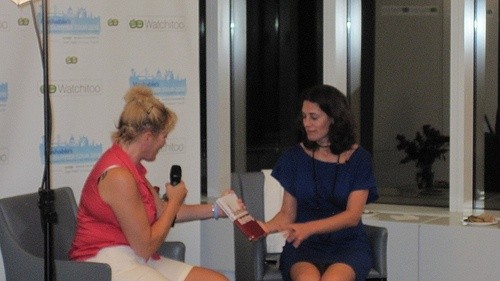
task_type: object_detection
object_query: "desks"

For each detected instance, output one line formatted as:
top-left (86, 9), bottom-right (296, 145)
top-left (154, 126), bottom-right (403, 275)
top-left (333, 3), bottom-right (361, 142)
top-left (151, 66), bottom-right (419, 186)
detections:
top-left (360, 210), bottom-right (500, 281)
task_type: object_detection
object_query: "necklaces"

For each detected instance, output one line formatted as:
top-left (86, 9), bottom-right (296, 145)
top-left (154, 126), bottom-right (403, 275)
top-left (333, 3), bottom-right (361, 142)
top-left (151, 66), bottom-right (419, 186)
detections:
top-left (312, 146), bottom-right (341, 208)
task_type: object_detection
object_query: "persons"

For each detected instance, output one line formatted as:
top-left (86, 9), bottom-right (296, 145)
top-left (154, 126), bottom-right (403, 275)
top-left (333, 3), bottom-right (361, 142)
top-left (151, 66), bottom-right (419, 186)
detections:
top-left (67, 85), bottom-right (246, 281)
top-left (254, 85), bottom-right (380, 281)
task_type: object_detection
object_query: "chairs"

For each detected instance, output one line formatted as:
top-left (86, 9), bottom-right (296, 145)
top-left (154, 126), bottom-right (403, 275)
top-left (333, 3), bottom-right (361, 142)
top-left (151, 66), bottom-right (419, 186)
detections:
top-left (231, 169), bottom-right (388, 281)
top-left (0, 186), bottom-right (186, 281)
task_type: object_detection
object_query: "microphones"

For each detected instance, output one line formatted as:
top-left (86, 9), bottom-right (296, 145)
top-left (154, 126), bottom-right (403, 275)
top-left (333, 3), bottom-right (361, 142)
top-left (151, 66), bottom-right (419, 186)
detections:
top-left (169, 165), bottom-right (182, 227)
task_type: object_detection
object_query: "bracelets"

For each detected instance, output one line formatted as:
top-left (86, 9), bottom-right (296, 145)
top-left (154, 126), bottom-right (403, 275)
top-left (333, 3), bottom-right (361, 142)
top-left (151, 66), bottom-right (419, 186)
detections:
top-left (213, 205), bottom-right (219, 219)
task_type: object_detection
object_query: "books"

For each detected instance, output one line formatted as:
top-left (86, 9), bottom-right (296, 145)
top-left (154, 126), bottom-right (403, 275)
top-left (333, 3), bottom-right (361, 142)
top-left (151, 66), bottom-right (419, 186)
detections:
top-left (216, 193), bottom-right (266, 242)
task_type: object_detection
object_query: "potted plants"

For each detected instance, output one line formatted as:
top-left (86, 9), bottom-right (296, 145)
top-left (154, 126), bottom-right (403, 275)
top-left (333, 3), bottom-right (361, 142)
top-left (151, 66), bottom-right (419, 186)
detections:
top-left (396, 125), bottom-right (450, 188)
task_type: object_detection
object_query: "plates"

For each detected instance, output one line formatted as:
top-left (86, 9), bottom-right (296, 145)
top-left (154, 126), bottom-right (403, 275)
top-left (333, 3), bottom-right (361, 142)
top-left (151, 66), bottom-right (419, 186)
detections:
top-left (461, 218), bottom-right (498, 225)
top-left (362, 211), bottom-right (377, 216)
